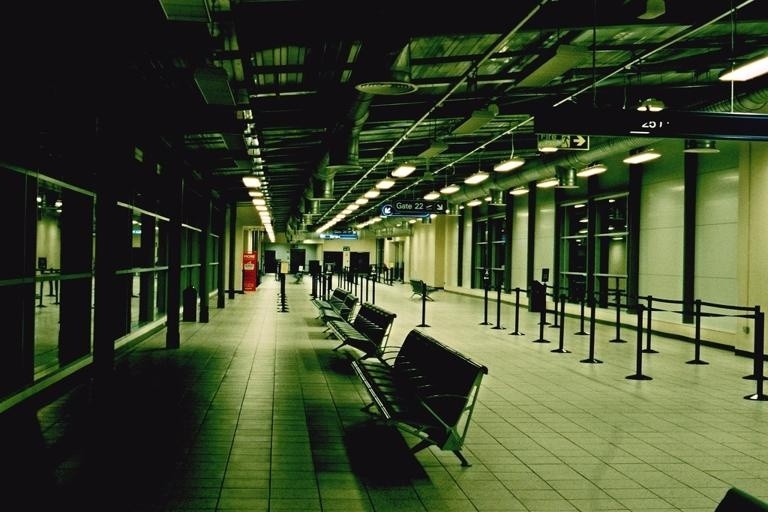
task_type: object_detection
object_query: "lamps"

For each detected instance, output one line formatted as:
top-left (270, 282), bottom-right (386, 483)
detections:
top-left (718, 49), bottom-right (768, 83)
top-left (249, 189), bottom-right (264, 197)
top-left (622, 150), bottom-right (662, 165)
top-left (464, 172), bottom-right (489, 185)
top-left (375, 177), bottom-right (396, 190)
top-left (424, 190), bottom-right (441, 201)
top-left (252, 198), bottom-right (276, 242)
top-left (509, 187), bottom-right (531, 196)
top-left (493, 157), bottom-right (525, 173)
top-left (537, 134), bottom-right (558, 152)
top-left (391, 163), bottom-right (417, 179)
top-left (242, 176), bottom-right (261, 188)
top-left (636, 98), bottom-right (666, 112)
top-left (439, 184), bottom-right (461, 195)
top-left (316, 189), bottom-right (381, 234)
top-left (575, 164), bottom-right (607, 178)
top-left (536, 178), bottom-right (560, 188)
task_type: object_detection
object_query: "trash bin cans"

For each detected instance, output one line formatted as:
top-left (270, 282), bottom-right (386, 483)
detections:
top-left (183, 285), bottom-right (197, 322)
top-left (532, 280), bottom-right (547, 312)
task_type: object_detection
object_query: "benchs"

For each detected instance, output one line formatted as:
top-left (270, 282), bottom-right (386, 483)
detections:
top-left (312, 279), bottom-right (489, 469)
